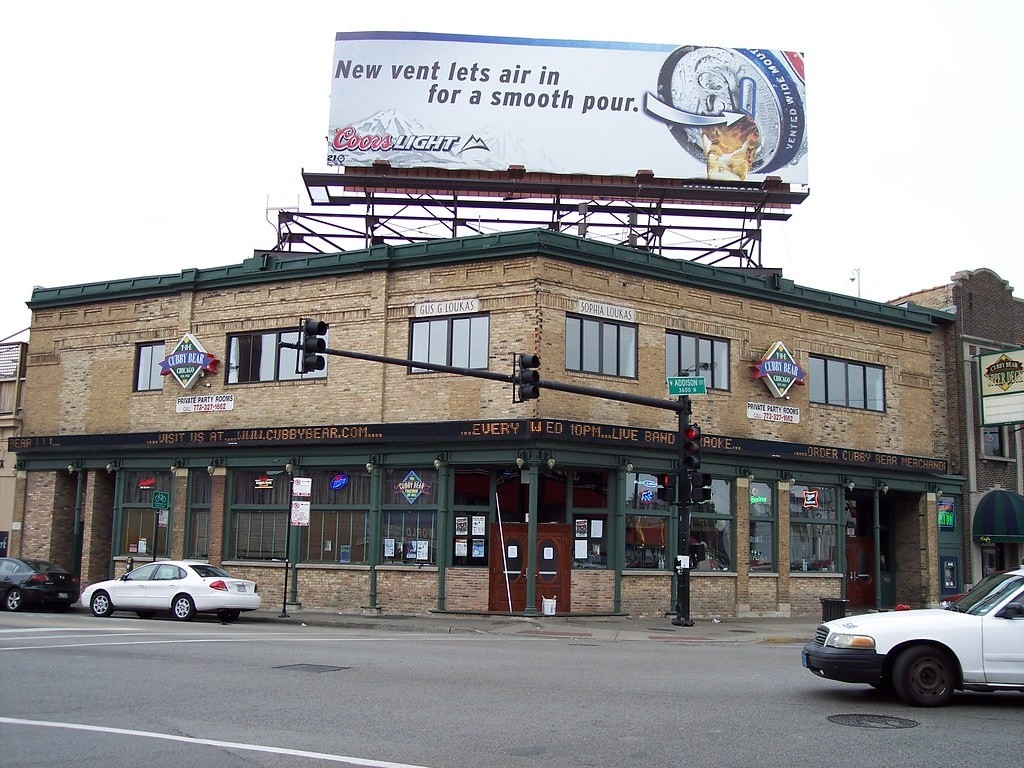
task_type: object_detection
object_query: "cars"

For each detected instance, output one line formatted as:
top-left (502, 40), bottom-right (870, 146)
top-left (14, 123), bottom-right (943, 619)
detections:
top-left (0, 557), bottom-right (80, 613)
top-left (79, 560), bottom-right (262, 622)
top-left (800, 565), bottom-right (1024, 707)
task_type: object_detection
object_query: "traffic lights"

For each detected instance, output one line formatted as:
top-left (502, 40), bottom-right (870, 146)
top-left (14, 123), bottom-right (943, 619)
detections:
top-left (295, 317), bottom-right (325, 374)
top-left (683, 424), bottom-right (702, 470)
top-left (689, 473), bottom-right (714, 504)
top-left (513, 352), bottom-right (540, 403)
top-left (655, 472), bottom-right (677, 503)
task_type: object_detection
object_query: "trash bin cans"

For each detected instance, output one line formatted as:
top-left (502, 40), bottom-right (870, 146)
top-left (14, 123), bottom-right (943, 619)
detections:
top-left (819, 598), bottom-right (850, 623)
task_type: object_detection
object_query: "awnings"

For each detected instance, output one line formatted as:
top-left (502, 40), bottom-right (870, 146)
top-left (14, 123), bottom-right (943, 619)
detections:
top-left (972, 490), bottom-right (1024, 544)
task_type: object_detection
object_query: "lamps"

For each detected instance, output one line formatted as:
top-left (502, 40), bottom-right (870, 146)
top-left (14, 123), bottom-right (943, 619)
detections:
top-left (106, 460), bottom-right (118, 474)
top-left (372, 158), bottom-right (393, 176)
top-left (11, 463), bottom-right (23, 476)
top-left (365, 457), bottom-right (377, 474)
top-left (286, 457), bottom-right (297, 476)
top-left (170, 458), bottom-right (182, 476)
top-left (743, 468), bottom-right (754, 484)
top-left (434, 453), bottom-right (445, 470)
top-left (925, 482), bottom-right (942, 499)
top-left (785, 473), bottom-right (796, 488)
top-left (207, 457), bottom-right (219, 477)
top-left (634, 169), bottom-right (655, 184)
top-left (843, 476), bottom-right (856, 491)
top-left (544, 450), bottom-right (556, 469)
top-left (506, 164), bottom-right (526, 181)
top-left (878, 480), bottom-right (889, 494)
top-left (622, 456), bottom-right (634, 474)
top-left (516, 451), bottom-right (529, 469)
top-left (67, 460), bottom-right (80, 474)
top-left (762, 177), bottom-right (783, 190)
